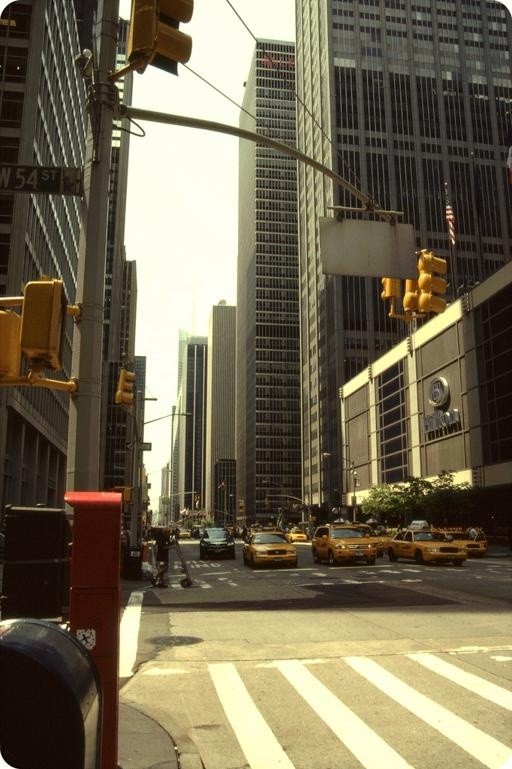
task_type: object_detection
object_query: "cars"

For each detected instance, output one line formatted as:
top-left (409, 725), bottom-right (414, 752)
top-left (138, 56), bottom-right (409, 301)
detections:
top-left (311, 520), bottom-right (377, 567)
top-left (348, 522), bottom-right (385, 559)
top-left (198, 527), bottom-right (236, 560)
top-left (284, 526), bottom-right (307, 544)
top-left (381, 520), bottom-right (468, 567)
top-left (242, 531), bottom-right (297, 569)
top-left (437, 531), bottom-right (486, 558)
top-left (173, 525), bottom-right (282, 544)
top-left (372, 528), bottom-right (393, 558)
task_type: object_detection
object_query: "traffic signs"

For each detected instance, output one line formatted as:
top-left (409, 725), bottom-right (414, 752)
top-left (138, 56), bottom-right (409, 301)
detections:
top-left (126, 442), bottom-right (152, 452)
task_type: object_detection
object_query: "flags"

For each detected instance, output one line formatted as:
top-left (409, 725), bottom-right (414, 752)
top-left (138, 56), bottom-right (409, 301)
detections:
top-left (445, 189), bottom-right (457, 245)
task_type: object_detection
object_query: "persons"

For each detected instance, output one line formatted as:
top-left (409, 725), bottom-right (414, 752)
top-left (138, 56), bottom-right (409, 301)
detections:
top-left (153, 527), bottom-right (174, 587)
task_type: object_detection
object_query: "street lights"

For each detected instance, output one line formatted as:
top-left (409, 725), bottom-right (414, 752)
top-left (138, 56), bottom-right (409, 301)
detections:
top-left (323, 452), bottom-right (356, 522)
top-left (139, 412), bottom-right (193, 551)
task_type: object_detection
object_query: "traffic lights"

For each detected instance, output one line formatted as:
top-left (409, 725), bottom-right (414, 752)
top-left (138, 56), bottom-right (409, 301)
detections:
top-left (419, 252), bottom-right (447, 316)
top-left (123, 487), bottom-right (132, 503)
top-left (115, 368), bottom-right (136, 404)
top-left (125, 0), bottom-right (194, 76)
top-left (20, 280), bottom-right (68, 372)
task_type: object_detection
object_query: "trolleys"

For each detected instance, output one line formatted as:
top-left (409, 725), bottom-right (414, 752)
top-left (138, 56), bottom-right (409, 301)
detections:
top-left (174, 541), bottom-right (200, 588)
